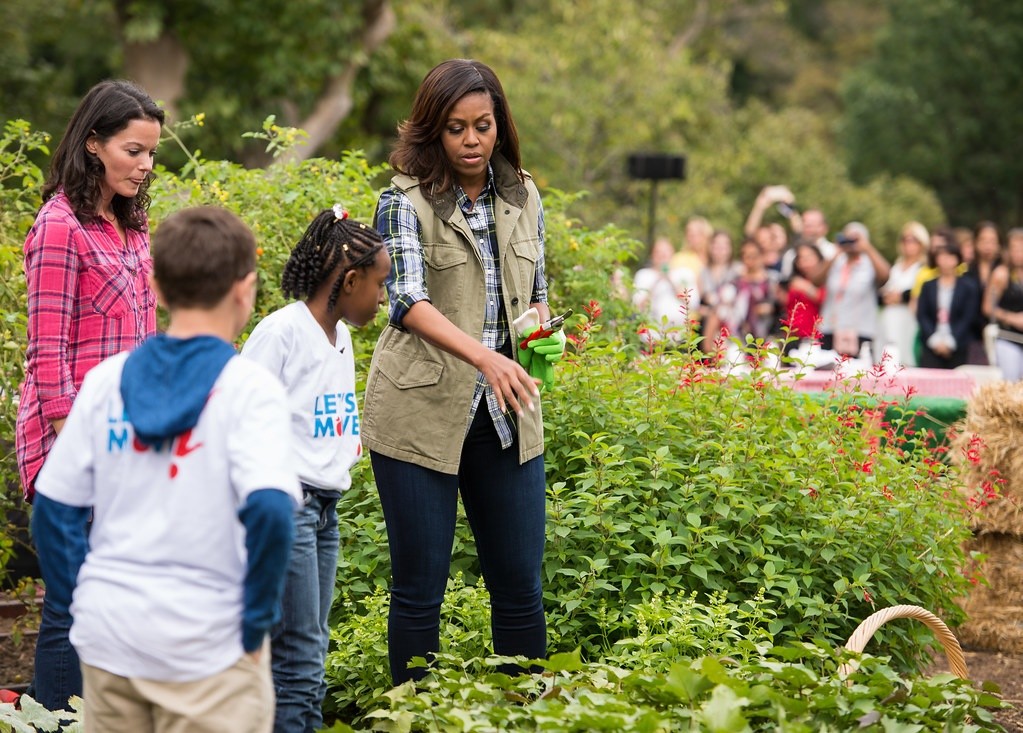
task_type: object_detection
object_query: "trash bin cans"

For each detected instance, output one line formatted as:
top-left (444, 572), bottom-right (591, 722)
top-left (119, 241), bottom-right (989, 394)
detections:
top-left (794, 392), bottom-right (872, 442)
top-left (861, 397), bottom-right (968, 467)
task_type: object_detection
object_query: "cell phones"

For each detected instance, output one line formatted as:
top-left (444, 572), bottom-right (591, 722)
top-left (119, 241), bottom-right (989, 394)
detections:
top-left (776, 203), bottom-right (794, 219)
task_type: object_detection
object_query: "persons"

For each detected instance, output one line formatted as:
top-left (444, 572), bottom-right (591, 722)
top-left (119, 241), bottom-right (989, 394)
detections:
top-left (361, 61), bottom-right (568, 706)
top-left (29, 204), bottom-right (305, 733)
top-left (575, 183), bottom-right (1023, 389)
top-left (15, 77), bottom-right (166, 733)
top-left (240, 209), bottom-right (391, 733)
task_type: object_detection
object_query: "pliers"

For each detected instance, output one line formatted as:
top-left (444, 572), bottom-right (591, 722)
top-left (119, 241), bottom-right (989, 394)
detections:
top-left (520, 308), bottom-right (573, 351)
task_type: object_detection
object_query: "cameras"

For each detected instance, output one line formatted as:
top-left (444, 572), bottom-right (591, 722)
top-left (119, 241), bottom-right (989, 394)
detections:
top-left (837, 233), bottom-right (855, 245)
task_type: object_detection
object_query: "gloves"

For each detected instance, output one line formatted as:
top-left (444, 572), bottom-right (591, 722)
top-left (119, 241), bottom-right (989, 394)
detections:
top-left (522, 319), bottom-right (566, 363)
top-left (512, 308), bottom-right (556, 393)
